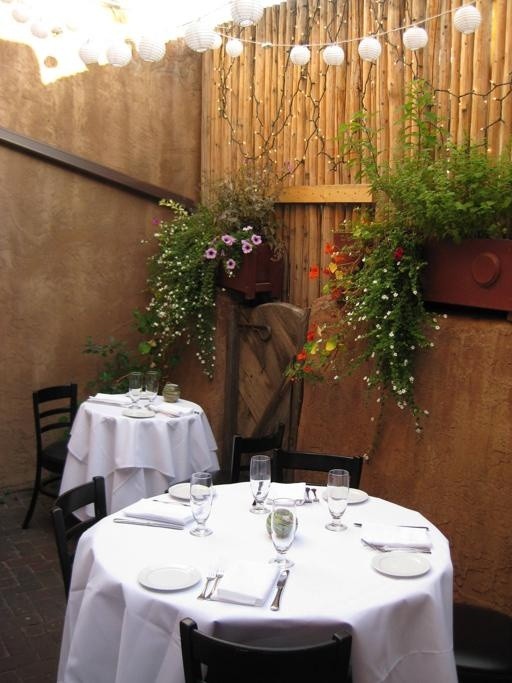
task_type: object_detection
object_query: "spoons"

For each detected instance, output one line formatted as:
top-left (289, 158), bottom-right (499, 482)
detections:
top-left (204, 569), bottom-right (224, 599)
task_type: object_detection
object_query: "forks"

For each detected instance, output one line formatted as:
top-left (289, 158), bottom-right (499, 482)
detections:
top-left (199, 571), bottom-right (214, 598)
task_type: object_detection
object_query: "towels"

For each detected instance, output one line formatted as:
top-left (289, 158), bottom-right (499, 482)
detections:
top-left (126, 498), bottom-right (194, 526)
top-left (88, 393), bottom-right (139, 407)
top-left (363, 523), bottom-right (432, 553)
top-left (210, 557), bottom-right (279, 606)
top-left (147, 399), bottom-right (192, 417)
top-left (266, 481), bottom-right (308, 508)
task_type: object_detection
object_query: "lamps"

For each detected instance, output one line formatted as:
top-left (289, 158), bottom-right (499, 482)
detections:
top-left (0, 0), bottom-right (481, 66)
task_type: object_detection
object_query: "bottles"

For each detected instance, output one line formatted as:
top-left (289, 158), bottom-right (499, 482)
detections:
top-left (164, 384), bottom-right (180, 403)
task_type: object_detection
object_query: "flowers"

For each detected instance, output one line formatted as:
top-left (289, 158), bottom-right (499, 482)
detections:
top-left (287, 75), bottom-right (512, 434)
top-left (145, 159), bottom-right (296, 381)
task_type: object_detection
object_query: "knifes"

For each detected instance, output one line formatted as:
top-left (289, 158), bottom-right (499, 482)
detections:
top-left (114, 519), bottom-right (182, 530)
top-left (272, 569), bottom-right (288, 609)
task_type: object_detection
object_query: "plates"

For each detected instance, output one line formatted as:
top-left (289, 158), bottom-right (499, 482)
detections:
top-left (373, 551), bottom-right (431, 576)
top-left (138, 564), bottom-right (200, 590)
top-left (322, 484), bottom-right (367, 502)
top-left (169, 481), bottom-right (213, 499)
top-left (123, 410), bottom-right (154, 419)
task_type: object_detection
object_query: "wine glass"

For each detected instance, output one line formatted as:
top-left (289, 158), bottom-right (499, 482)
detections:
top-left (129, 372), bottom-right (142, 409)
top-left (325, 469), bottom-right (350, 530)
top-left (146, 371), bottom-right (158, 407)
top-left (190, 472), bottom-right (213, 536)
top-left (271, 499), bottom-right (297, 564)
top-left (250, 455), bottom-right (269, 513)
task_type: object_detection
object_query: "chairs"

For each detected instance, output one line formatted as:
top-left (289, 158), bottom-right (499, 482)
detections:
top-left (21, 382), bottom-right (77, 529)
top-left (453, 602), bottom-right (512, 680)
top-left (52, 476), bottom-right (107, 605)
top-left (272, 449), bottom-right (363, 488)
top-left (230, 422), bottom-right (285, 483)
top-left (180, 615), bottom-right (353, 683)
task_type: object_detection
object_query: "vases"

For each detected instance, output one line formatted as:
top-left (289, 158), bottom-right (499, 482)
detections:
top-left (412, 237), bottom-right (512, 321)
top-left (217, 245), bottom-right (282, 303)
